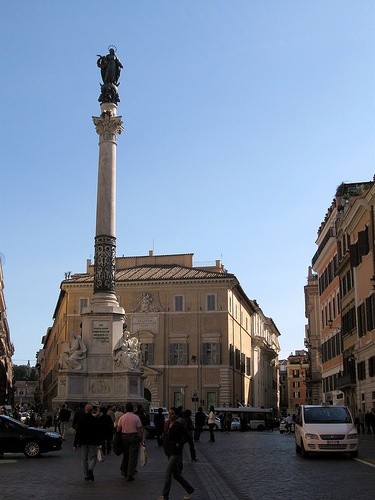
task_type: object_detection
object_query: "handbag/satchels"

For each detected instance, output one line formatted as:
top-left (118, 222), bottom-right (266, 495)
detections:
top-left (95, 446), bottom-right (105, 462)
top-left (139, 446), bottom-right (147, 467)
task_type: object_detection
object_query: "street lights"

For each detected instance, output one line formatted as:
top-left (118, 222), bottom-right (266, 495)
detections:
top-left (305, 340), bottom-right (321, 350)
top-left (328, 319), bottom-right (352, 336)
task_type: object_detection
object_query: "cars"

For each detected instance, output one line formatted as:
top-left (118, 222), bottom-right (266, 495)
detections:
top-left (295, 404), bottom-right (358, 458)
top-left (149, 408), bottom-right (169, 421)
top-left (280, 420), bottom-right (294, 434)
top-left (0, 414), bottom-right (62, 457)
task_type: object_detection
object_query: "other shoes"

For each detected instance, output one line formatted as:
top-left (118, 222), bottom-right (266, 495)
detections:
top-left (62, 438), bottom-right (65, 441)
top-left (208, 438), bottom-right (215, 442)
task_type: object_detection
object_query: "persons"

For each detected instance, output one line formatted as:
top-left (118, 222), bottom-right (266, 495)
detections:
top-left (96, 45), bottom-right (123, 87)
top-left (354, 408), bottom-right (375, 435)
top-left (0, 402), bottom-right (295, 500)
top-left (112, 332), bottom-right (141, 371)
top-left (58, 335), bottom-right (87, 369)
top-left (139, 292), bottom-right (150, 313)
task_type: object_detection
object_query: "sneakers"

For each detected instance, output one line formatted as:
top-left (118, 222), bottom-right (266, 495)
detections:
top-left (121, 469), bottom-right (138, 481)
top-left (158, 496), bottom-right (168, 500)
top-left (184, 490), bottom-right (194, 500)
top-left (85, 469), bottom-right (94, 481)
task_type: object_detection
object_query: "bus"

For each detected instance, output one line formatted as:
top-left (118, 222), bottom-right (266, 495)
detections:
top-left (207, 407), bottom-right (274, 431)
